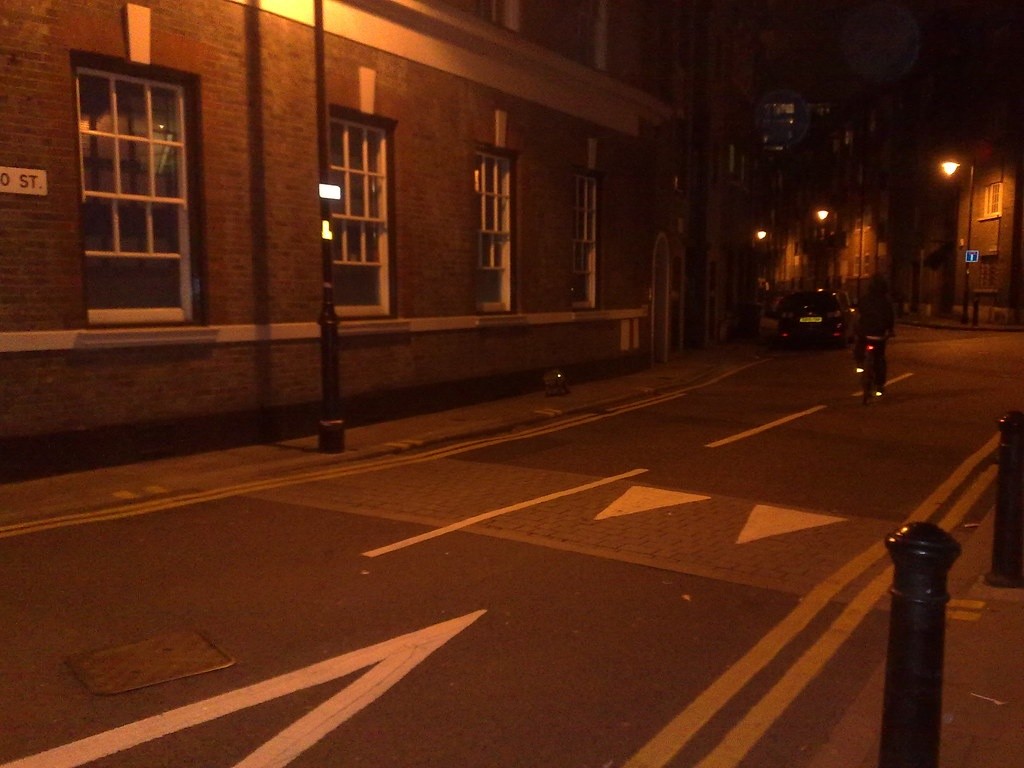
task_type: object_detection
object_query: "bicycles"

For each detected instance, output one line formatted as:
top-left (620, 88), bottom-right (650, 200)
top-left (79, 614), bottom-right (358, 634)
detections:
top-left (855, 333), bottom-right (884, 406)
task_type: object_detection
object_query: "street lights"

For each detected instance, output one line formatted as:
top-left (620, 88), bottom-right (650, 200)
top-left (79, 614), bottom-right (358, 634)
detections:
top-left (757, 226), bottom-right (775, 296)
top-left (815, 206), bottom-right (838, 288)
top-left (939, 159), bottom-right (974, 323)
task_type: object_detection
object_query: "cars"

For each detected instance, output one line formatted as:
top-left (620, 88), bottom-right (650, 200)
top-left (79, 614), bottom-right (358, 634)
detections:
top-left (776, 289), bottom-right (857, 348)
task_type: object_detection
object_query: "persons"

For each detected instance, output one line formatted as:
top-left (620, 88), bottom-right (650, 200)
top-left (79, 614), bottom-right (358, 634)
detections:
top-left (790, 271), bottom-right (842, 290)
top-left (857, 274), bottom-right (895, 393)
